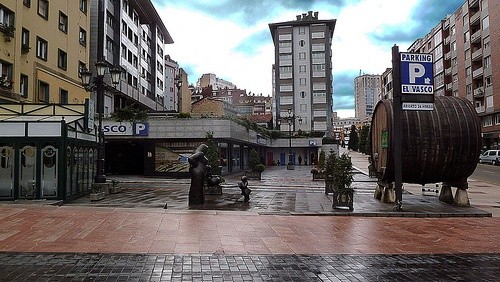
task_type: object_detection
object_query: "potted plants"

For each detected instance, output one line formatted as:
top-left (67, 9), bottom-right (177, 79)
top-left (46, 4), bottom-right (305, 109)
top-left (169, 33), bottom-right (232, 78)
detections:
top-left (90, 180), bottom-right (121, 201)
top-left (205, 131), bottom-right (225, 194)
top-left (368, 164), bottom-right (377, 178)
top-left (245, 148), bottom-right (265, 180)
top-left (313, 148), bottom-right (355, 211)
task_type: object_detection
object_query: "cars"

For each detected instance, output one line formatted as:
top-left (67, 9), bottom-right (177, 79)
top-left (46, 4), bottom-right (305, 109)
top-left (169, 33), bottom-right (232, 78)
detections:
top-left (478, 150), bottom-right (500, 166)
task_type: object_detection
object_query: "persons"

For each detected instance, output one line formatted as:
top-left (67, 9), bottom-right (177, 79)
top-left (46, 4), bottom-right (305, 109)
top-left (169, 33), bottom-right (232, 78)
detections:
top-left (298, 155), bottom-right (302, 165)
top-left (238, 176), bottom-right (251, 203)
top-left (187, 144), bottom-right (208, 204)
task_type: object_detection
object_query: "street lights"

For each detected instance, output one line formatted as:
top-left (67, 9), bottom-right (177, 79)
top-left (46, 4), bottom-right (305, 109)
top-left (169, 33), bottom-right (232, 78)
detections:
top-left (81, 56), bottom-right (123, 196)
top-left (277, 107), bottom-right (303, 170)
